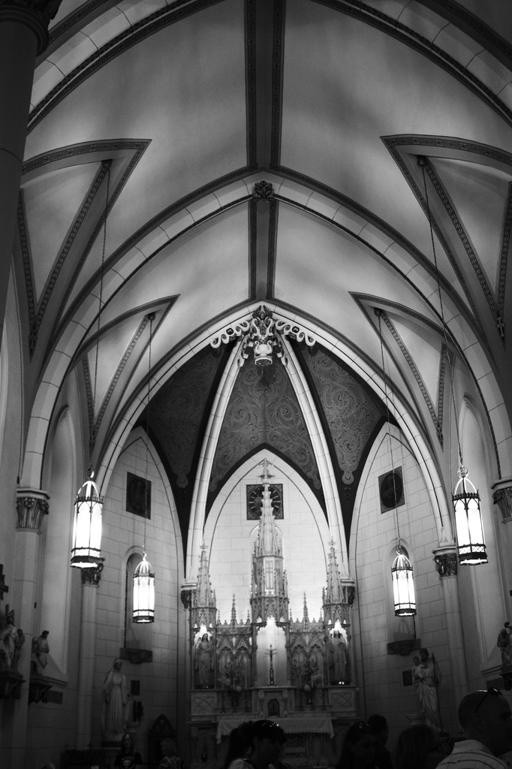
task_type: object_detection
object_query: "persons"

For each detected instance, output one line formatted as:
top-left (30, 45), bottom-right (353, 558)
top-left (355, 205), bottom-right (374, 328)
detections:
top-left (115, 734), bottom-right (144, 768)
top-left (410, 648), bottom-right (444, 733)
top-left (197, 633), bottom-right (213, 689)
top-left (335, 713), bottom-right (439, 768)
top-left (497, 621), bottom-right (512, 690)
top-left (159, 738), bottom-right (183, 769)
top-left (311, 665), bottom-right (323, 704)
top-left (32, 629), bottom-right (49, 674)
top-left (225, 720), bottom-right (287, 769)
top-left (220, 672), bottom-right (236, 710)
top-left (102, 659), bottom-right (131, 732)
top-left (10, 628), bottom-right (25, 669)
top-left (411, 656), bottom-right (426, 680)
top-left (437, 692), bottom-right (511, 768)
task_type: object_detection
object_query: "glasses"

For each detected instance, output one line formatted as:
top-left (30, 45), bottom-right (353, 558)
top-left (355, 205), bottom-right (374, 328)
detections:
top-left (355, 720), bottom-right (376, 729)
top-left (262, 721), bottom-right (277, 728)
top-left (473, 686), bottom-right (502, 711)
top-left (426, 743), bottom-right (444, 753)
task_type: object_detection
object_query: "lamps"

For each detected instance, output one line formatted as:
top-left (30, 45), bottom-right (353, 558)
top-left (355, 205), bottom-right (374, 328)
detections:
top-left (69, 160), bottom-right (110, 570)
top-left (417, 154), bottom-right (487, 567)
top-left (376, 308), bottom-right (418, 616)
top-left (133, 316), bottom-right (157, 625)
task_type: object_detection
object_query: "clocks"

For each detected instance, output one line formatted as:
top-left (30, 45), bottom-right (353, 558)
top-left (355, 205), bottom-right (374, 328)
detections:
top-left (247, 484), bottom-right (284, 519)
